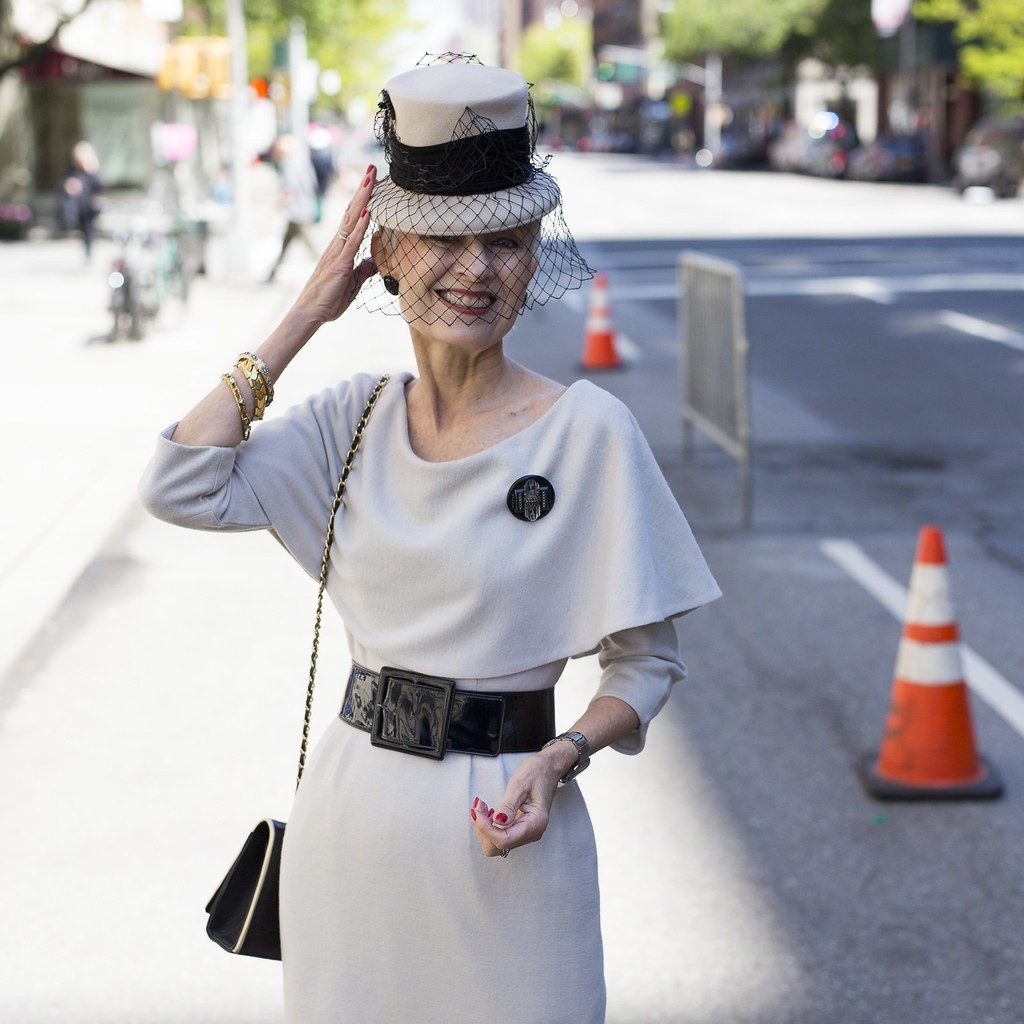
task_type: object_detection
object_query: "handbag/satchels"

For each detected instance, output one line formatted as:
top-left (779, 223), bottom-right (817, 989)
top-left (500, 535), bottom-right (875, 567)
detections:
top-left (205, 818), bottom-right (286, 960)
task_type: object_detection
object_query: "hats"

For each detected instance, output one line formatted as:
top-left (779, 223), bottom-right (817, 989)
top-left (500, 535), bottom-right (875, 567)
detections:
top-left (348, 51), bottom-right (598, 327)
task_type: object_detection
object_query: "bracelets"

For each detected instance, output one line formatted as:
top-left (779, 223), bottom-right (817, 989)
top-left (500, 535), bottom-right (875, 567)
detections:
top-left (222, 351), bottom-right (274, 442)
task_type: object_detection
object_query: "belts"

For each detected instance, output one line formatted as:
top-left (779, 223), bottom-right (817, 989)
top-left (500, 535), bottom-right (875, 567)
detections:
top-left (338, 659), bottom-right (556, 760)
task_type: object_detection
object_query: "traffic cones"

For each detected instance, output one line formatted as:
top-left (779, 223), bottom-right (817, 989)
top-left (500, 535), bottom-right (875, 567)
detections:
top-left (850, 525), bottom-right (1007, 802)
top-left (576, 273), bottom-right (637, 376)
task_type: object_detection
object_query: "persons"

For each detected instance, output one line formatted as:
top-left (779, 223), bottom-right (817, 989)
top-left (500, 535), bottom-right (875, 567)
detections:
top-left (140, 51), bottom-right (723, 1024)
top-left (63, 142), bottom-right (105, 257)
top-left (254, 120), bottom-right (336, 289)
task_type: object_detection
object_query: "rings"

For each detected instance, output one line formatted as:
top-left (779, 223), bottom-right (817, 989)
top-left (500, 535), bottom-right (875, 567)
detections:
top-left (338, 228), bottom-right (349, 240)
top-left (501, 849), bottom-right (510, 858)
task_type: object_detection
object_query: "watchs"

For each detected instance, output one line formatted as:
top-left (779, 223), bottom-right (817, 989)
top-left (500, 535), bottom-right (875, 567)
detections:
top-left (542, 732), bottom-right (591, 783)
